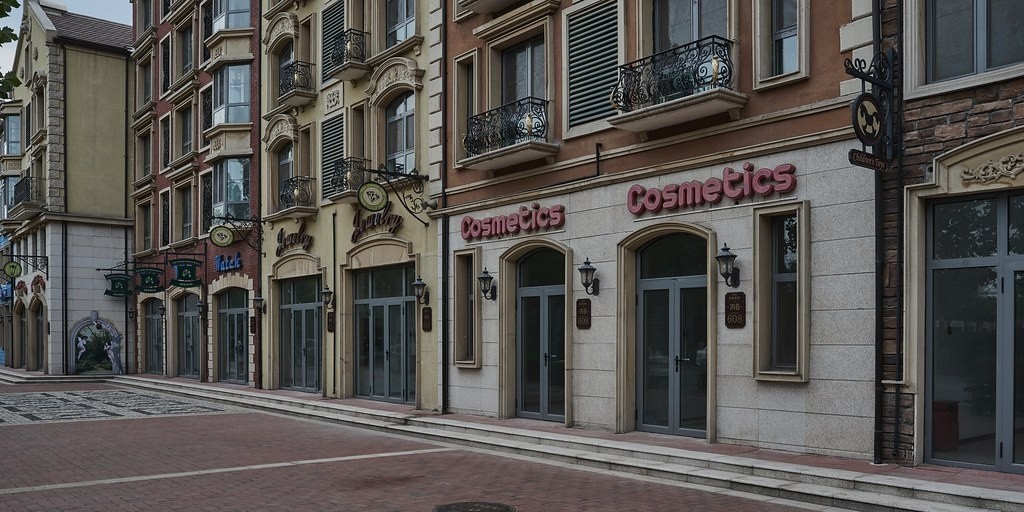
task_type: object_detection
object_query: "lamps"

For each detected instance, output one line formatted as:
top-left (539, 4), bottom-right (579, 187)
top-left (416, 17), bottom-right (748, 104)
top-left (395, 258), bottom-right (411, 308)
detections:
top-left (714, 242), bottom-right (740, 288)
top-left (128, 308), bottom-right (138, 322)
top-left (252, 292), bottom-right (266, 315)
top-left (0, 314), bottom-right (4, 326)
top-left (577, 257), bottom-right (600, 296)
top-left (477, 267), bottom-right (497, 301)
top-left (321, 284), bottom-right (336, 310)
top-left (196, 300), bottom-right (207, 319)
top-left (412, 274), bottom-right (429, 305)
top-left (6, 312), bottom-right (13, 325)
top-left (158, 304), bottom-right (168, 323)
